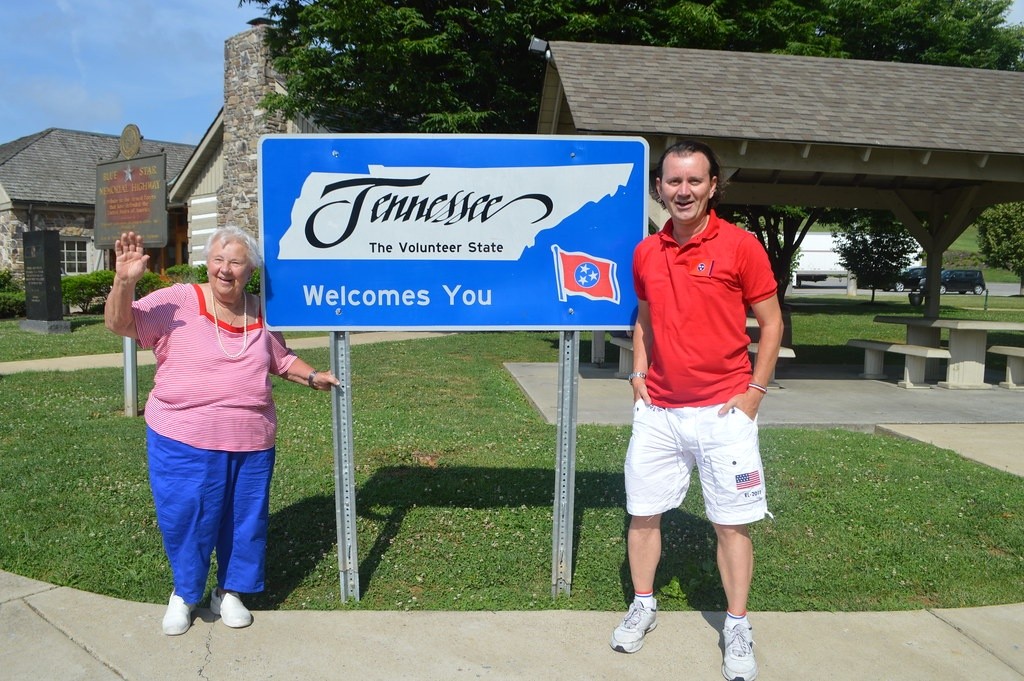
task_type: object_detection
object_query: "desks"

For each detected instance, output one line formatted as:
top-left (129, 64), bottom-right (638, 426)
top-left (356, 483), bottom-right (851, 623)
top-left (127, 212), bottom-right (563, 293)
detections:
top-left (873, 315), bottom-right (1024, 390)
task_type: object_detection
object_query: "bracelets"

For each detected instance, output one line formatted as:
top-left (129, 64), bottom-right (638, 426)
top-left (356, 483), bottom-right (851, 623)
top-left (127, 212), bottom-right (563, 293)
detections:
top-left (309, 370), bottom-right (319, 390)
top-left (748, 382), bottom-right (767, 393)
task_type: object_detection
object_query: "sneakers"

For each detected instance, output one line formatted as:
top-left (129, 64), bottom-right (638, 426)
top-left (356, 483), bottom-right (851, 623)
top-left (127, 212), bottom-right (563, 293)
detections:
top-left (162, 587), bottom-right (196, 635)
top-left (610, 598), bottom-right (657, 653)
top-left (722, 618), bottom-right (760, 681)
top-left (210, 587), bottom-right (253, 628)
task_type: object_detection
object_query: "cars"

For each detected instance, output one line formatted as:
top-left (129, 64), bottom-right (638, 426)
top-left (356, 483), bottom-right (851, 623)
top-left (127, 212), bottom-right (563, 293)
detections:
top-left (881, 266), bottom-right (945, 292)
top-left (918, 269), bottom-right (986, 294)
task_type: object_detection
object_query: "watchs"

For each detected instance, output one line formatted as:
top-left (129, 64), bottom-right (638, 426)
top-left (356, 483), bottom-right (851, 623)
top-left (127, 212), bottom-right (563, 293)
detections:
top-left (628, 372), bottom-right (647, 385)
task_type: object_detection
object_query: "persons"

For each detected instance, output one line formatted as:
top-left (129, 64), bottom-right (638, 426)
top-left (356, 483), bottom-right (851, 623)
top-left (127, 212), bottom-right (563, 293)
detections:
top-left (613, 140), bottom-right (785, 681)
top-left (104, 224), bottom-right (340, 636)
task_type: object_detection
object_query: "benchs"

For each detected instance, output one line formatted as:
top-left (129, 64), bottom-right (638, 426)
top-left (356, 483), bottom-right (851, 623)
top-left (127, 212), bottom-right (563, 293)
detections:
top-left (791, 269), bottom-right (848, 287)
top-left (608, 337), bottom-right (635, 373)
top-left (746, 342), bottom-right (795, 384)
top-left (987, 345), bottom-right (1024, 389)
top-left (846, 338), bottom-right (951, 389)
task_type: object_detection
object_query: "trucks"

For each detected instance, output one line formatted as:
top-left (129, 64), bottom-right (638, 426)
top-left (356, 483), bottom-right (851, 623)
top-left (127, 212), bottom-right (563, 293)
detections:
top-left (752, 231), bottom-right (925, 287)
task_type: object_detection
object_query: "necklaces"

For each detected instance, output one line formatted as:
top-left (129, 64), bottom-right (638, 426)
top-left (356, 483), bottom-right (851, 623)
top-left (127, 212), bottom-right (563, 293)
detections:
top-left (214, 299), bottom-right (242, 326)
top-left (209, 283), bottom-right (247, 359)
